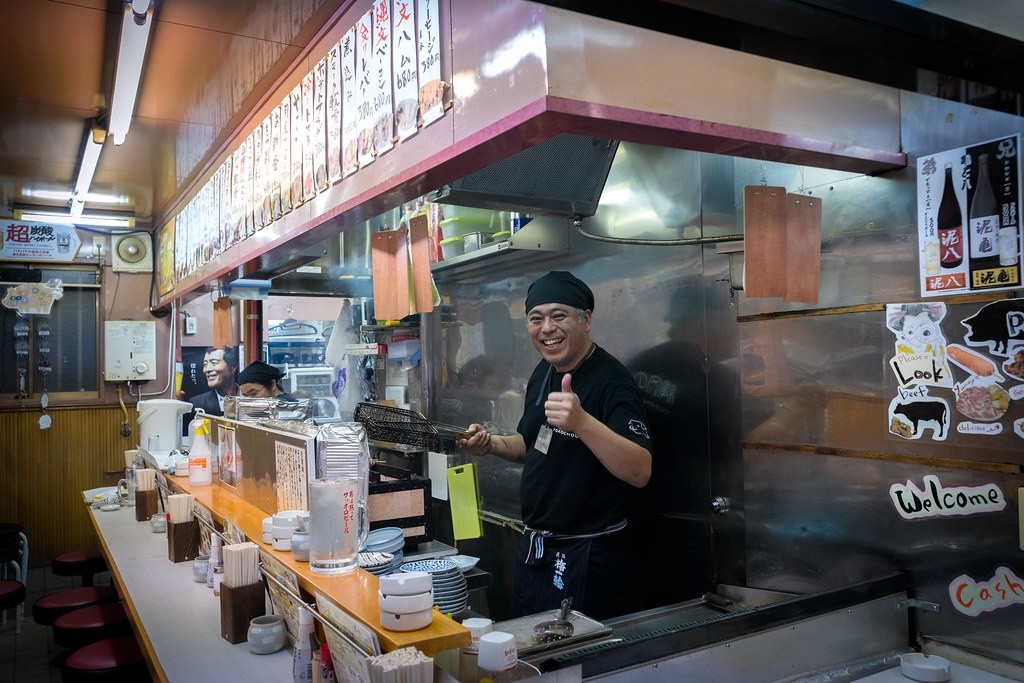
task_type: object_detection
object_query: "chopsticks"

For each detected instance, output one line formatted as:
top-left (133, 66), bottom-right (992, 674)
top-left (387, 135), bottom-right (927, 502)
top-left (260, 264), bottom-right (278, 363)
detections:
top-left (167, 494), bottom-right (195, 523)
top-left (222, 542), bottom-right (260, 587)
top-left (366, 646), bottom-right (434, 683)
top-left (136, 469), bottom-right (155, 491)
top-left (125, 450), bottom-right (139, 467)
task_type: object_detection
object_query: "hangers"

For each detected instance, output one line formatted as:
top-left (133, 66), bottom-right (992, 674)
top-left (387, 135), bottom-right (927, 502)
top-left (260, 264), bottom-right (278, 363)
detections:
top-left (267, 305), bottom-right (317, 336)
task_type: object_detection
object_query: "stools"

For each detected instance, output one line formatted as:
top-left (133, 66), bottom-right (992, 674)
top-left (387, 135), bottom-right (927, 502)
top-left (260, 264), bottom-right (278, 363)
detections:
top-left (51, 547), bottom-right (109, 587)
top-left (61, 634), bottom-right (155, 683)
top-left (32, 585), bottom-right (119, 626)
top-left (0, 523), bottom-right (29, 634)
top-left (53, 602), bottom-right (134, 649)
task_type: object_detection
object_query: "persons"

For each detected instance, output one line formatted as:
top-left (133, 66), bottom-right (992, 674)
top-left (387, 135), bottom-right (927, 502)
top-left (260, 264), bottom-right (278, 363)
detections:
top-left (187, 344), bottom-right (240, 419)
top-left (237, 360), bottom-right (295, 404)
top-left (453, 271), bottom-right (653, 624)
top-left (618, 276), bottom-right (826, 608)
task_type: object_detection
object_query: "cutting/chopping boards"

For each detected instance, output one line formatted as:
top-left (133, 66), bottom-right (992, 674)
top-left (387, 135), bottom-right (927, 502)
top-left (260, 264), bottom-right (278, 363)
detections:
top-left (447, 463), bottom-right (481, 540)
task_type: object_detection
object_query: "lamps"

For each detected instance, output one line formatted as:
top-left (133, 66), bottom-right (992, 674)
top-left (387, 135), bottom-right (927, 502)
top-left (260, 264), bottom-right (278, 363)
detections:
top-left (15, 185), bottom-right (136, 206)
top-left (70, 118), bottom-right (107, 216)
top-left (14, 208), bottom-right (135, 229)
top-left (108, 0), bottom-right (160, 146)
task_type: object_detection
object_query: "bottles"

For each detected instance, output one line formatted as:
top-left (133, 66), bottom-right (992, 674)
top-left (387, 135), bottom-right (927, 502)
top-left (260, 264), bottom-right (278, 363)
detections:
top-left (312, 650), bottom-right (321, 683)
top-left (207, 533), bottom-right (223, 588)
top-left (320, 644), bottom-right (335, 683)
top-left (969, 155), bottom-right (999, 268)
top-left (213, 566), bottom-right (224, 596)
top-left (938, 162), bottom-right (963, 268)
top-left (292, 607), bottom-right (320, 683)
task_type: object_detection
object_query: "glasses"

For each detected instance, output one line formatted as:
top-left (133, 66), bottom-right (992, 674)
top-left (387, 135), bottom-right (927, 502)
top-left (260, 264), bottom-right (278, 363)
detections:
top-left (244, 385), bottom-right (263, 397)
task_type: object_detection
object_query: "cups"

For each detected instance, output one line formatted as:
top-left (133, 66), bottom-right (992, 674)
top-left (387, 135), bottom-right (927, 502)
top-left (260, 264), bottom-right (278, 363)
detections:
top-left (117, 466), bottom-right (144, 508)
top-left (999, 226), bottom-right (1022, 265)
top-left (310, 478), bottom-right (369, 573)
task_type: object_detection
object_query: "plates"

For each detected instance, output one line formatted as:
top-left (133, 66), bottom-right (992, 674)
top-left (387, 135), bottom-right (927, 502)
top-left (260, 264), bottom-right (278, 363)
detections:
top-left (84, 498), bottom-right (104, 502)
top-left (100, 505), bottom-right (120, 510)
top-left (357, 527), bottom-right (405, 576)
top-left (400, 555), bottom-right (480, 616)
